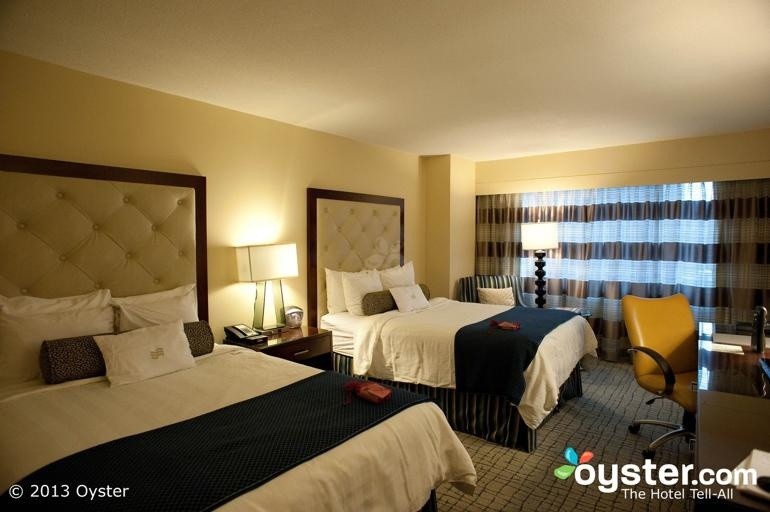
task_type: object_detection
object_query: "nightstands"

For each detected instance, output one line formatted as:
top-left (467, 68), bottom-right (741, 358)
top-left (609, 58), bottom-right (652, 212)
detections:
top-left (223, 325), bottom-right (333, 372)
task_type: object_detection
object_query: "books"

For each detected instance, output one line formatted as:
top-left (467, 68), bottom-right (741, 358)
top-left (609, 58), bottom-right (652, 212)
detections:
top-left (720, 448), bottom-right (770, 502)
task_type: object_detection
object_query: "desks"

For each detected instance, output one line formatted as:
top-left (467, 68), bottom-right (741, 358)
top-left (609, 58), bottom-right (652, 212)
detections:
top-left (696, 322), bottom-right (770, 512)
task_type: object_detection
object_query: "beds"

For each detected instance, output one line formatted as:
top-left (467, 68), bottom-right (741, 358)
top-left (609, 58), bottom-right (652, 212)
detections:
top-left (0, 153), bottom-right (477, 512)
top-left (307, 187), bottom-right (600, 452)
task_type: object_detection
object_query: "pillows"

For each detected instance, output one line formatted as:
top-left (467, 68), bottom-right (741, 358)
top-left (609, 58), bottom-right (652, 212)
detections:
top-left (324, 261), bottom-right (430, 314)
top-left (0, 283), bottom-right (215, 388)
top-left (477, 287), bottom-right (516, 306)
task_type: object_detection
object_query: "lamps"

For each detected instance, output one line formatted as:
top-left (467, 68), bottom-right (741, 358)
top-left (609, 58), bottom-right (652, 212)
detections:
top-left (235, 242), bottom-right (299, 334)
top-left (520, 222), bottom-right (559, 309)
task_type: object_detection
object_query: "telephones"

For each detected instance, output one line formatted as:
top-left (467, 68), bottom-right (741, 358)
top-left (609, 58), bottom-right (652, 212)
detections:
top-left (224, 324), bottom-right (268, 344)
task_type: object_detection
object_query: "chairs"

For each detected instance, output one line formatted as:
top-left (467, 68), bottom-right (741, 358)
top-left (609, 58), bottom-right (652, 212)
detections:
top-left (458, 275), bottom-right (529, 307)
top-left (622, 293), bottom-right (697, 460)
